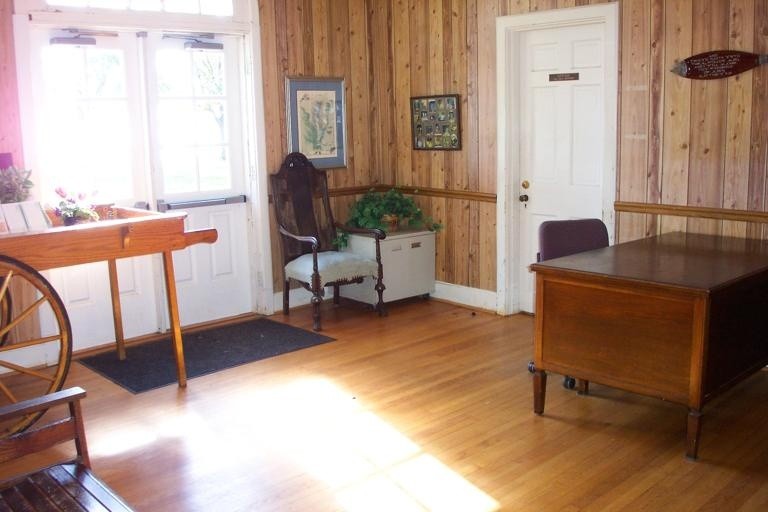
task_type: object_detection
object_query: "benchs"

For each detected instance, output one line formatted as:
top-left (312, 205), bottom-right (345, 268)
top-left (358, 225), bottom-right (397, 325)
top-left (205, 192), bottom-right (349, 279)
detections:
top-left (0, 386), bottom-right (137, 512)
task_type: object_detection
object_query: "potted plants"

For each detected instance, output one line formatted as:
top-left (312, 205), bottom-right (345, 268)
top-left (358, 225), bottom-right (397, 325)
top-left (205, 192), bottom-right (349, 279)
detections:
top-left (332, 186), bottom-right (444, 247)
top-left (0, 164), bottom-right (47, 233)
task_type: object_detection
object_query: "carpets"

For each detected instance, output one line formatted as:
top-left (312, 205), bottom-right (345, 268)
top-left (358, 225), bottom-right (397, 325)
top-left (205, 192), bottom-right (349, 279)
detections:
top-left (77, 318), bottom-right (338, 393)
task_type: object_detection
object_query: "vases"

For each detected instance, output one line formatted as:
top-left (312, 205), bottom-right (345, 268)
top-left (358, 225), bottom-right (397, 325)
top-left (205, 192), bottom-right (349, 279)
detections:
top-left (64, 216), bottom-right (81, 226)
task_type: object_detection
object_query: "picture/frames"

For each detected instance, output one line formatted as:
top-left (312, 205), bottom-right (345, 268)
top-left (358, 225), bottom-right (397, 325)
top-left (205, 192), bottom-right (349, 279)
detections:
top-left (285, 75), bottom-right (350, 171)
top-left (408, 93), bottom-right (462, 151)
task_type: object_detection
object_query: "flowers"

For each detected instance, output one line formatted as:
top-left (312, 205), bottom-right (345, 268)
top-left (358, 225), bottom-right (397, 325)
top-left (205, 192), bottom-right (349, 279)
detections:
top-left (53, 185), bottom-right (101, 227)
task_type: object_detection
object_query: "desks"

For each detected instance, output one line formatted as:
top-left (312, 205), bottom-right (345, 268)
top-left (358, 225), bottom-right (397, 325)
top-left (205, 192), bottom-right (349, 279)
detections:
top-left (332, 230), bottom-right (437, 309)
top-left (528, 231), bottom-right (767, 461)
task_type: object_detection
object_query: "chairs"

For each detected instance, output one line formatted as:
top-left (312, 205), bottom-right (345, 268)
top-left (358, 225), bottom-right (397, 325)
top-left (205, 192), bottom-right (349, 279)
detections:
top-left (269, 151), bottom-right (386, 331)
top-left (527, 220), bottom-right (610, 388)
